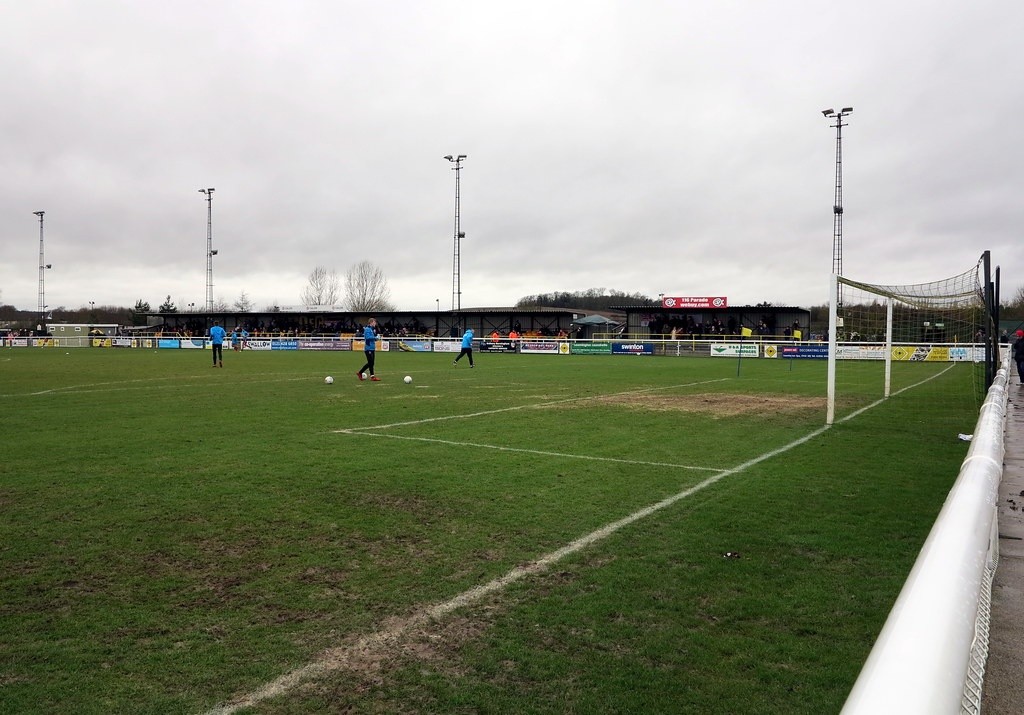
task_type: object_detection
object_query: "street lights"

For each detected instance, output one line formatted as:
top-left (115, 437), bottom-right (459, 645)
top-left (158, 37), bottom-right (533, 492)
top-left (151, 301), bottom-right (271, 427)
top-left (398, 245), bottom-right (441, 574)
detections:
top-left (436, 298), bottom-right (439, 311)
top-left (187, 302), bottom-right (194, 314)
top-left (89, 301), bottom-right (95, 311)
top-left (443, 154), bottom-right (466, 337)
top-left (32, 210), bottom-right (51, 331)
top-left (821, 107), bottom-right (854, 332)
top-left (197, 187), bottom-right (218, 337)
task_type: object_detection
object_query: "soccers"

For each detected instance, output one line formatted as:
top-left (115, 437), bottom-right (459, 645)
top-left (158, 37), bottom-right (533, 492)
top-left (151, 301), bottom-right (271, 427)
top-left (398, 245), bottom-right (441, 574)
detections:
top-left (154, 351), bottom-right (157, 353)
top-left (325, 376), bottom-right (334, 384)
top-left (362, 373), bottom-right (367, 379)
top-left (404, 376), bottom-right (413, 383)
top-left (66, 352), bottom-right (69, 355)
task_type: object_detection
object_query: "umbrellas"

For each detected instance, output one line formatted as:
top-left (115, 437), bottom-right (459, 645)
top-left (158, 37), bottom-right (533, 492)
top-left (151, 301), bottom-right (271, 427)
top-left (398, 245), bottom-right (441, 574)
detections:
top-left (571, 314), bottom-right (618, 332)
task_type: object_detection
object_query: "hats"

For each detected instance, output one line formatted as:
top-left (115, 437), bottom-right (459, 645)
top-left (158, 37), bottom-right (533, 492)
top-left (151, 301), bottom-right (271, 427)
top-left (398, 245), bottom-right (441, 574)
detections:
top-left (1016, 330), bottom-right (1024, 337)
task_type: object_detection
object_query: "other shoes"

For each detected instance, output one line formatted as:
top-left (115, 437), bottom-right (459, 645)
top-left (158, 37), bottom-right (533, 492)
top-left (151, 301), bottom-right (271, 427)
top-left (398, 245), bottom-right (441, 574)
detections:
top-left (219, 362), bottom-right (222, 367)
top-left (470, 364), bottom-right (475, 368)
top-left (454, 361), bottom-right (457, 364)
top-left (357, 372), bottom-right (363, 381)
top-left (1016, 382), bottom-right (1024, 385)
top-left (212, 365), bottom-right (217, 367)
top-left (370, 377), bottom-right (380, 381)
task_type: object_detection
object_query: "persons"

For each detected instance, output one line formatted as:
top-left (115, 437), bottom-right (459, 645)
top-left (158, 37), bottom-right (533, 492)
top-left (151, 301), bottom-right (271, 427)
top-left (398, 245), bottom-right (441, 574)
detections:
top-left (783, 320), bottom-right (800, 341)
top-left (648, 314), bottom-right (770, 340)
top-left (8, 332), bottom-right (14, 347)
top-left (88, 330), bottom-right (107, 336)
top-left (254, 322), bottom-right (354, 340)
top-left (382, 321), bottom-right (436, 341)
top-left (357, 318), bottom-right (381, 381)
top-left (836, 328), bottom-right (884, 346)
top-left (115, 326), bottom-right (140, 336)
top-left (228, 329), bottom-right (252, 352)
top-left (356, 330), bottom-right (363, 340)
top-left (210, 321), bottom-right (225, 367)
top-left (975, 329), bottom-right (1024, 385)
top-left (536, 326), bottom-right (584, 341)
top-left (492, 320), bottom-right (521, 343)
top-left (453, 328), bottom-right (475, 368)
top-left (160, 319), bottom-right (205, 337)
